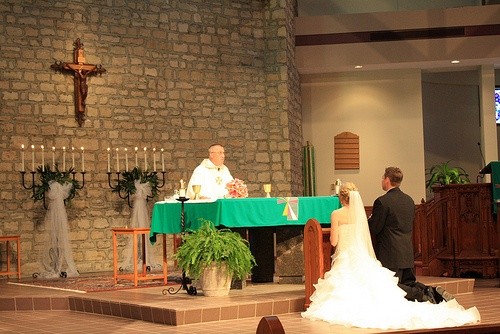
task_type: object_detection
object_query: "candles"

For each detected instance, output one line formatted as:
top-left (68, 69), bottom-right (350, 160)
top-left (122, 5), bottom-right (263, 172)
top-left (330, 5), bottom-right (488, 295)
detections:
top-left (115, 147), bottom-right (120, 172)
top-left (134, 146), bottom-right (139, 167)
top-left (21, 142), bottom-right (25, 171)
top-left (124, 147), bottom-right (129, 172)
top-left (160, 147), bottom-right (165, 172)
top-left (143, 146), bottom-right (148, 170)
top-left (80, 146), bottom-right (85, 172)
top-left (41, 145), bottom-right (45, 171)
top-left (52, 146), bottom-right (56, 171)
top-left (71, 147), bottom-right (76, 171)
top-left (178, 187), bottom-right (185, 197)
top-left (106, 148), bottom-right (111, 172)
top-left (63, 146), bottom-right (66, 171)
top-left (31, 144), bottom-right (35, 171)
top-left (153, 147), bottom-right (157, 171)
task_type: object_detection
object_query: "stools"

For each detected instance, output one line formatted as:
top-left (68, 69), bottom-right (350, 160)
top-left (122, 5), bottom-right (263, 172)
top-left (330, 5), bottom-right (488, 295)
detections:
top-left (0, 234), bottom-right (22, 280)
top-left (110, 225), bottom-right (169, 287)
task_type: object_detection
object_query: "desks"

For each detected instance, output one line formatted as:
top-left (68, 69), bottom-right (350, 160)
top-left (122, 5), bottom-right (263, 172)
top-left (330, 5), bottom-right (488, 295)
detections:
top-left (147, 196), bottom-right (342, 289)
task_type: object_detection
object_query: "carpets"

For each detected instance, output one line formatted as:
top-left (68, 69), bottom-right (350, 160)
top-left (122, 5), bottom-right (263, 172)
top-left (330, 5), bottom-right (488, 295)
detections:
top-left (6, 273), bottom-right (194, 294)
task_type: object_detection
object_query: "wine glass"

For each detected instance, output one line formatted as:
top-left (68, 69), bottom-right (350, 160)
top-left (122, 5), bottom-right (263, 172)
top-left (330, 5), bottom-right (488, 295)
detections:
top-left (263, 183), bottom-right (272, 198)
top-left (192, 185), bottom-right (201, 200)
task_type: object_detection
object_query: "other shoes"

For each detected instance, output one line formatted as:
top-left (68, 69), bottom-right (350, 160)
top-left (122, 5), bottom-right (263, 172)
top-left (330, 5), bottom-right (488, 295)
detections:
top-left (436, 286), bottom-right (455, 302)
top-left (423, 286), bottom-right (440, 304)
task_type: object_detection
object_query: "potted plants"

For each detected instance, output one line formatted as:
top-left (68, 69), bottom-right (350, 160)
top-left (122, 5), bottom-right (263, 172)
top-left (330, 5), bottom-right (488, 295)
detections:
top-left (172, 217), bottom-right (256, 298)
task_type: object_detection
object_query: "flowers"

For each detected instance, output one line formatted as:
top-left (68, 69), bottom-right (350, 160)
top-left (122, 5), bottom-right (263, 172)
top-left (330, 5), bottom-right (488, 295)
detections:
top-left (224, 176), bottom-right (251, 198)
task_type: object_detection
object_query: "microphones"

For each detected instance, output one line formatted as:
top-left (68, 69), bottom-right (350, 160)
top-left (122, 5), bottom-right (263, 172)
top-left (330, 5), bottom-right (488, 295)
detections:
top-left (478, 142), bottom-right (486, 166)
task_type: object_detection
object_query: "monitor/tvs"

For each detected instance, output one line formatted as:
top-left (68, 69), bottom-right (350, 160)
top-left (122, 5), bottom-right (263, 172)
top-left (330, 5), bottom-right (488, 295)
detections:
top-left (495, 88), bottom-right (500, 125)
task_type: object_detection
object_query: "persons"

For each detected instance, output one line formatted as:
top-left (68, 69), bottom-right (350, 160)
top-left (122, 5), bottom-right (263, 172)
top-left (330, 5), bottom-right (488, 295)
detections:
top-left (185, 144), bottom-right (237, 201)
top-left (301, 181), bottom-right (480, 330)
top-left (366, 166), bottom-right (458, 304)
